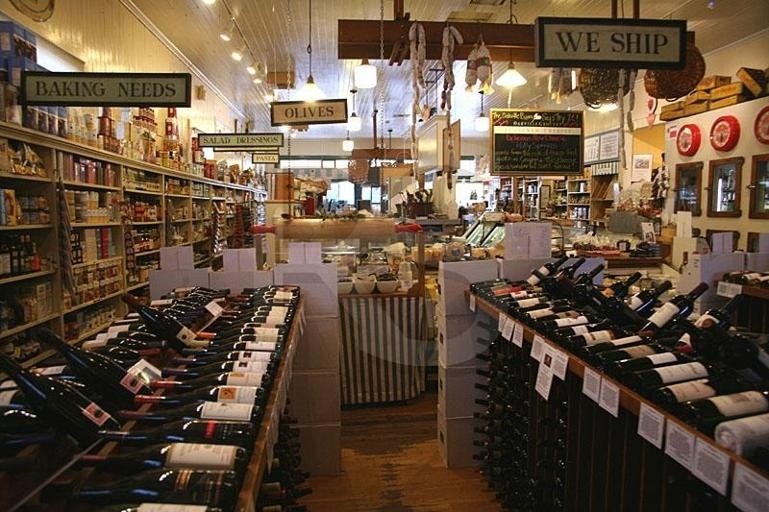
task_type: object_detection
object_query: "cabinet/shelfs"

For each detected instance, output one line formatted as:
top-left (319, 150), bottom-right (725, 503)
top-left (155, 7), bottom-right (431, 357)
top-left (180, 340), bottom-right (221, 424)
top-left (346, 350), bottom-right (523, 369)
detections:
top-left (0, 12), bottom-right (308, 512)
top-left (337, 92), bottom-right (769, 512)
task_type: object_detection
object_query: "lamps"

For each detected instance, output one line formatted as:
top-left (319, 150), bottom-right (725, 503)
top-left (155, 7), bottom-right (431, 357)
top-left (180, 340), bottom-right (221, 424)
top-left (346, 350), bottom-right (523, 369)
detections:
top-left (220, 22), bottom-right (236, 43)
top-left (345, 89), bottom-right (364, 133)
top-left (230, 43), bottom-right (246, 62)
top-left (354, 58), bottom-right (378, 90)
top-left (246, 59), bottom-right (260, 73)
top-left (474, 91), bottom-right (491, 133)
top-left (252, 73), bottom-right (264, 84)
top-left (495, 2), bottom-right (527, 91)
top-left (341, 129), bottom-right (356, 153)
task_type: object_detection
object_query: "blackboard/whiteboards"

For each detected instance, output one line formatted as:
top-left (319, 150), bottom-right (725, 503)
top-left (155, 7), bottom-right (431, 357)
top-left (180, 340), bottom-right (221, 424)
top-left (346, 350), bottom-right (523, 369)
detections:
top-left (489, 107), bottom-right (584, 176)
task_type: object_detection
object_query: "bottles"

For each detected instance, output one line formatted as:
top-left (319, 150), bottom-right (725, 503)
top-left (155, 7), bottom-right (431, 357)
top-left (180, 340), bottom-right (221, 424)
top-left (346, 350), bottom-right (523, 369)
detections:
top-left (0, 232), bottom-right (41, 279)
top-left (50, 285), bottom-right (298, 512)
top-left (134, 228), bottom-right (160, 254)
top-left (397, 261), bottom-right (413, 290)
top-left (470, 255), bottom-right (769, 512)
top-left (0, 287), bottom-right (228, 512)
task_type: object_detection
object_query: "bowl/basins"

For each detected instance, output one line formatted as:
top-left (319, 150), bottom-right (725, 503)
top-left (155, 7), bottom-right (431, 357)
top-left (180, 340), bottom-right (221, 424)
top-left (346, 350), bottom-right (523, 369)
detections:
top-left (376, 276), bottom-right (399, 293)
top-left (337, 277), bottom-right (355, 295)
top-left (354, 276), bottom-right (377, 295)
